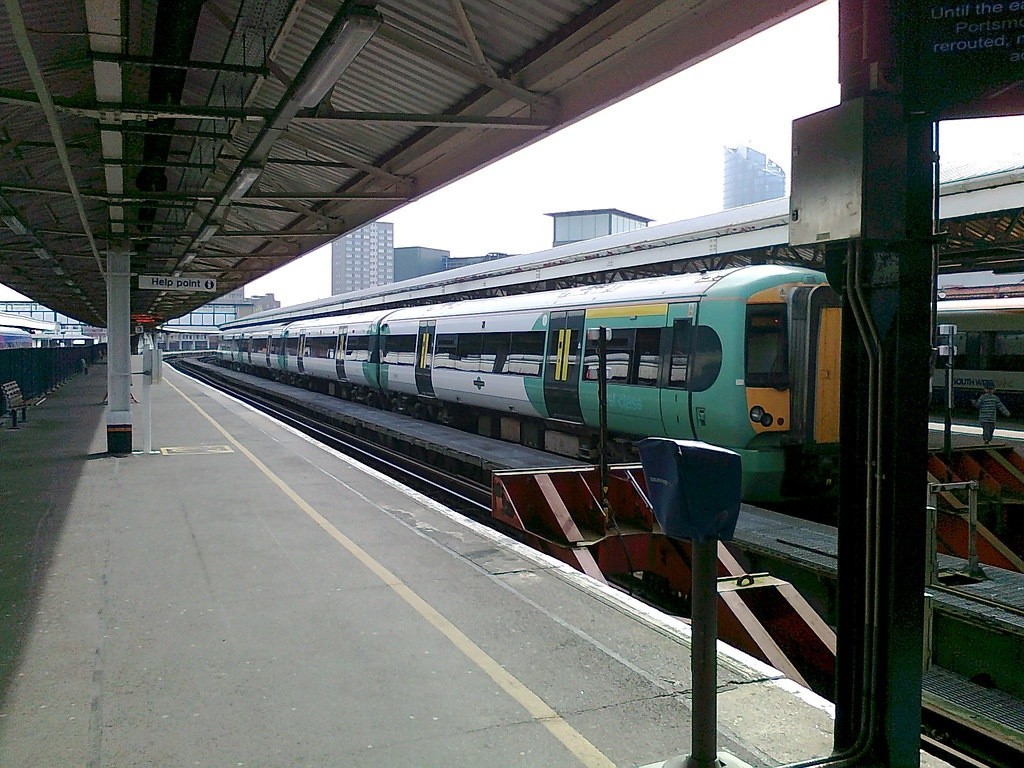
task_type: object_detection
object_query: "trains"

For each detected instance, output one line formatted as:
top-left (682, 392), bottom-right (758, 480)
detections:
top-left (214, 264), bottom-right (843, 512)
top-left (931, 297), bottom-right (1023, 417)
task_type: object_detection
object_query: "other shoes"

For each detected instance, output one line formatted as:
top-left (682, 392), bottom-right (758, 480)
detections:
top-left (989, 443), bottom-right (993, 445)
top-left (984, 440), bottom-right (989, 444)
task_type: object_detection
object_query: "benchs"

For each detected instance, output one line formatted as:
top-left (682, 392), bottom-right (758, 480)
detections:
top-left (1, 381), bottom-right (47, 430)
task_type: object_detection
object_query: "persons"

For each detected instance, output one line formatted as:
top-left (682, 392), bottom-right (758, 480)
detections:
top-left (972, 382), bottom-right (1010, 444)
